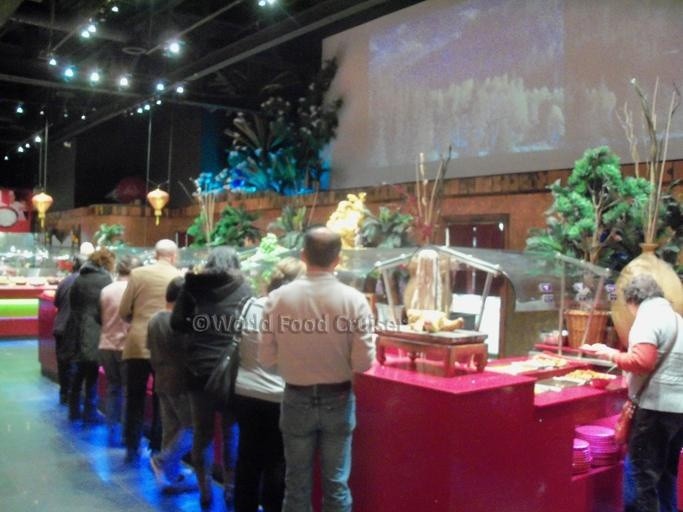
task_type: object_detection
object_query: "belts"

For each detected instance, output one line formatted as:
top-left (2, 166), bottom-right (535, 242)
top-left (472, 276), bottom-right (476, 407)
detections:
top-left (285, 380), bottom-right (351, 392)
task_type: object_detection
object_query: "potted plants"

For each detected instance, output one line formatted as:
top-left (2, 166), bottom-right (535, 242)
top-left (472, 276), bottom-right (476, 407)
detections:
top-left (527, 146), bottom-right (665, 350)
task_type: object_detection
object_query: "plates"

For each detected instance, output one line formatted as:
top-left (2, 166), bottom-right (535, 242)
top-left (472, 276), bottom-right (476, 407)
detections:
top-left (571, 425), bottom-right (618, 475)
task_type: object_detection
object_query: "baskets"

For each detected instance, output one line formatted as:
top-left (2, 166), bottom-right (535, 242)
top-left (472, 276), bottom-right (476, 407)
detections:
top-left (565, 309), bottom-right (609, 350)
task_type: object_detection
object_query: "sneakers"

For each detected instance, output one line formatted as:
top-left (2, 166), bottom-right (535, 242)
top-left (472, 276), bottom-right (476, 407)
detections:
top-left (61, 395), bottom-right (231, 510)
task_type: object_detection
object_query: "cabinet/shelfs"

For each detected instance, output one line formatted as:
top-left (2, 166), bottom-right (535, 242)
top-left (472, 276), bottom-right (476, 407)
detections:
top-left (0, 234), bottom-right (75, 338)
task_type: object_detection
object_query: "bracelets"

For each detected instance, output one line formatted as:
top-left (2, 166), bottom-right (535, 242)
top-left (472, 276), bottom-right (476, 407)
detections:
top-left (609, 349), bottom-right (618, 362)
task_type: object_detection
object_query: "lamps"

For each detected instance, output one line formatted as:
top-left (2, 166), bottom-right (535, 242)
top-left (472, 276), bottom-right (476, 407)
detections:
top-left (147, 185), bottom-right (169, 227)
top-left (29, 186), bottom-right (52, 226)
top-left (41, 0), bottom-right (283, 114)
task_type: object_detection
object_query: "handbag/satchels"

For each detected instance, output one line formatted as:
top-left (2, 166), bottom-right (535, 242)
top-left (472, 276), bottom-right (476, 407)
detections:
top-left (204, 337), bottom-right (253, 413)
top-left (614, 401), bottom-right (638, 444)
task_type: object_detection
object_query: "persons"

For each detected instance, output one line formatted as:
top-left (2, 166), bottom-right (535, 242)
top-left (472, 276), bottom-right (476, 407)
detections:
top-left (578, 274), bottom-right (682, 511)
